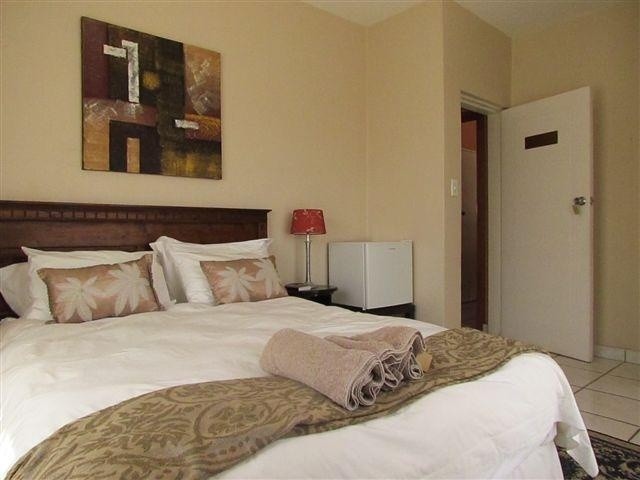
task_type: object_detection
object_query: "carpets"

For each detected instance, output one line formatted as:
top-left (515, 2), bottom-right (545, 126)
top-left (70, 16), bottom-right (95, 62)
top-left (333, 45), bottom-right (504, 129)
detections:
top-left (555, 430), bottom-right (640, 480)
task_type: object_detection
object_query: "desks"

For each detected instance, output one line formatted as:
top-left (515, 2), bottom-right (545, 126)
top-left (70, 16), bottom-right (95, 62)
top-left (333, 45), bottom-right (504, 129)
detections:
top-left (330, 303), bottom-right (415, 320)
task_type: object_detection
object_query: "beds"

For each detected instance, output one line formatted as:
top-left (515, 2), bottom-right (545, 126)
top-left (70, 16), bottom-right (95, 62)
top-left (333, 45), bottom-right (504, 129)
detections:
top-left (0, 201), bottom-right (601, 480)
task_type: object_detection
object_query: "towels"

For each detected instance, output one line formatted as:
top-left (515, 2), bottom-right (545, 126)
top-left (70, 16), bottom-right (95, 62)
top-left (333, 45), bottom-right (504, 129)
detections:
top-left (261, 326), bottom-right (428, 413)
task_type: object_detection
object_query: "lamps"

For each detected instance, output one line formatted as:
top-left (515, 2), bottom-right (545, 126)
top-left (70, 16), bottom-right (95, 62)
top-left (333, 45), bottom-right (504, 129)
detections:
top-left (289, 209), bottom-right (326, 289)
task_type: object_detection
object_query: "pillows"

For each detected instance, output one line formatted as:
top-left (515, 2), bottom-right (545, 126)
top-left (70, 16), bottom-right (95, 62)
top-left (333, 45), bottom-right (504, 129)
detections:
top-left (156, 235), bottom-right (275, 307)
top-left (0, 259), bottom-right (27, 318)
top-left (36, 253), bottom-right (167, 328)
top-left (20, 245), bottom-right (175, 323)
top-left (147, 239), bottom-right (186, 303)
top-left (198, 255), bottom-right (288, 307)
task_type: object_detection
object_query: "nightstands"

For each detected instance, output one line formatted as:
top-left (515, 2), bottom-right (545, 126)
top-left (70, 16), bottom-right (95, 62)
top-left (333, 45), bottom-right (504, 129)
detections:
top-left (284, 282), bottom-right (338, 306)
